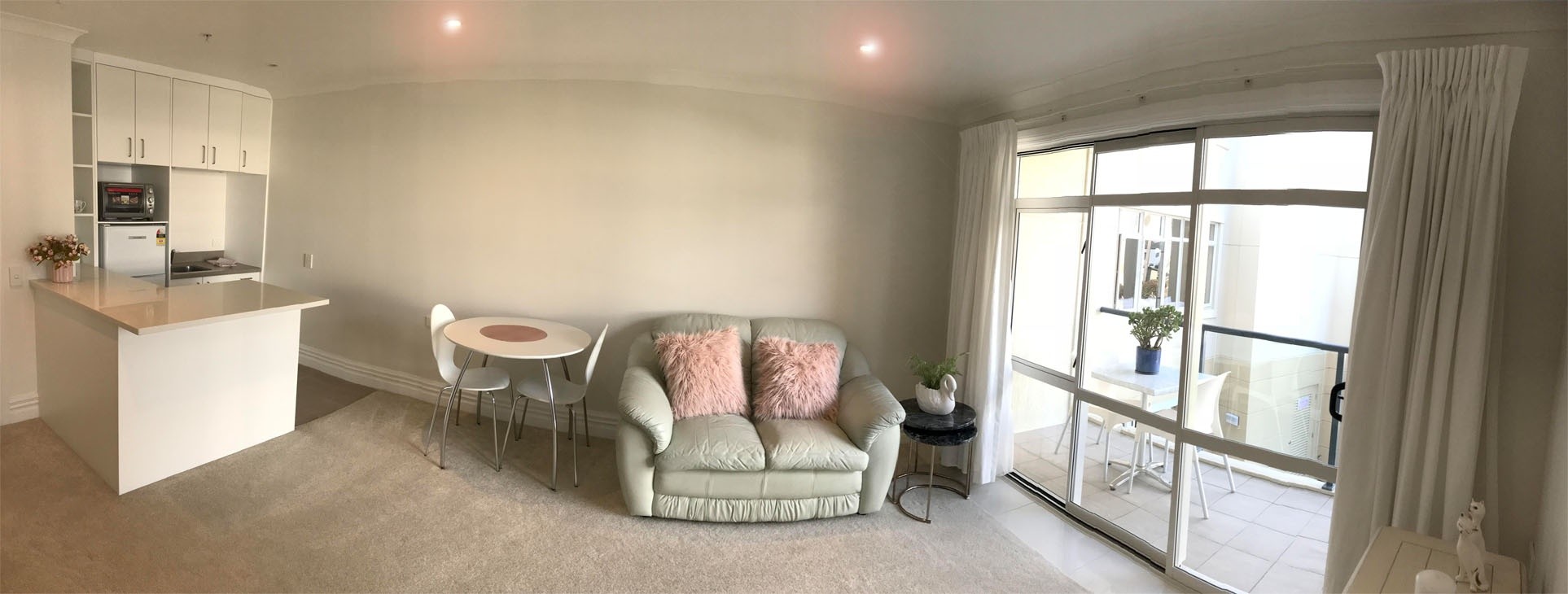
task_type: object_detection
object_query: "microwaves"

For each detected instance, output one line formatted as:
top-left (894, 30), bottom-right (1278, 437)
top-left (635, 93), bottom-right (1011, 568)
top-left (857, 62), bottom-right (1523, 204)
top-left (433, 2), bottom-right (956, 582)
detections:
top-left (98, 181), bottom-right (155, 221)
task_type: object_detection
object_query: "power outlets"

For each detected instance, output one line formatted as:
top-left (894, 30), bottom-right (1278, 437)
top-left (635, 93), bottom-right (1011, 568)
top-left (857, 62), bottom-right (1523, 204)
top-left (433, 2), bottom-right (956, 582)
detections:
top-left (305, 253), bottom-right (313, 269)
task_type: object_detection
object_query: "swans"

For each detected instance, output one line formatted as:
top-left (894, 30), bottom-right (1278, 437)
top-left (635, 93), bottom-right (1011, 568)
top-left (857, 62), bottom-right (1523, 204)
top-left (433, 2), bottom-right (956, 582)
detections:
top-left (915, 374), bottom-right (957, 415)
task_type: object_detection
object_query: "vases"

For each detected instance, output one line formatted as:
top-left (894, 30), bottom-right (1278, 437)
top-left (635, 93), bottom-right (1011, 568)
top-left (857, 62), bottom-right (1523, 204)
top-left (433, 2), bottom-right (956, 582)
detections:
top-left (52, 263), bottom-right (72, 284)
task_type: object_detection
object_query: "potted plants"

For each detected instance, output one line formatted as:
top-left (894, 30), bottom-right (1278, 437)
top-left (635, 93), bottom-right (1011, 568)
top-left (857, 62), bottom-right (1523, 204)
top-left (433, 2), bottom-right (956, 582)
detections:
top-left (908, 350), bottom-right (970, 416)
top-left (1128, 305), bottom-right (1183, 376)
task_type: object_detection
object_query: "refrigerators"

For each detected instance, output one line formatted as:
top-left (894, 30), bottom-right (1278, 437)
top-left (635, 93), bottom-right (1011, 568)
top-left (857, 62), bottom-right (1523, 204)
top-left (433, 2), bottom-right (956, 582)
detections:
top-left (96, 223), bottom-right (166, 288)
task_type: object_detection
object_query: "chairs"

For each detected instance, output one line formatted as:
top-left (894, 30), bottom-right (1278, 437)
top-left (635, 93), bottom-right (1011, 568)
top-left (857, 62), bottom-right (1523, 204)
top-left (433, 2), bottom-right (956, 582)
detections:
top-left (1054, 349), bottom-right (1154, 482)
top-left (499, 323), bottom-right (611, 487)
top-left (1127, 371), bottom-right (1236, 520)
top-left (424, 303), bottom-right (518, 473)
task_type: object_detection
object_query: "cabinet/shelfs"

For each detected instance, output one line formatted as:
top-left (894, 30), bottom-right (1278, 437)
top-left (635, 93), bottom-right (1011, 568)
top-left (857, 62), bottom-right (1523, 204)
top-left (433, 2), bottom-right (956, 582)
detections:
top-left (72, 51), bottom-right (273, 288)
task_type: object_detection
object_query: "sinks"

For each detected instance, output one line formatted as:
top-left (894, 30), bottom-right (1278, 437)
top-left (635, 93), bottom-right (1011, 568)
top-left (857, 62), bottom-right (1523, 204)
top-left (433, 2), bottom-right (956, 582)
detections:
top-left (171, 265), bottom-right (212, 273)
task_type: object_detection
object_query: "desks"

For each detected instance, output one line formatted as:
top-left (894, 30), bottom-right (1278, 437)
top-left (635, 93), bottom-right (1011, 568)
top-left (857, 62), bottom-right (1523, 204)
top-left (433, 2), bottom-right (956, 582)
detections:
top-left (1091, 363), bottom-right (1218, 490)
top-left (440, 316), bottom-right (591, 490)
top-left (1343, 525), bottom-right (1521, 594)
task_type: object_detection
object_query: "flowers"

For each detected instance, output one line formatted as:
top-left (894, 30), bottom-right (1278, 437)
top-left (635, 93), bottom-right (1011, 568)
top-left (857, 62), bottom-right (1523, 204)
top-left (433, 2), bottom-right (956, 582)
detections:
top-left (27, 234), bottom-right (90, 270)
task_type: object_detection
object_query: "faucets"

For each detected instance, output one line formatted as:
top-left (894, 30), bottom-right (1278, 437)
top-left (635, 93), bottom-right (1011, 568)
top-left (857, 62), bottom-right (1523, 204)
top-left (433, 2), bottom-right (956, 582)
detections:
top-left (171, 249), bottom-right (176, 266)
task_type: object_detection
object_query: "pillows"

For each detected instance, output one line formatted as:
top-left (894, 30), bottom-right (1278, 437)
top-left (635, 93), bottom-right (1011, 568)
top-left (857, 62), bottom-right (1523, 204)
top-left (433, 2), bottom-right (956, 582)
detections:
top-left (751, 336), bottom-right (844, 424)
top-left (655, 330), bottom-right (752, 424)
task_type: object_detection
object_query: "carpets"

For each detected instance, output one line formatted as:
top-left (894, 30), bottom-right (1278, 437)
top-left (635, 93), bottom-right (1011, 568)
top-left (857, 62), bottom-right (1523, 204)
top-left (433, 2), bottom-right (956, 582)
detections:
top-left (0, 388), bottom-right (1095, 594)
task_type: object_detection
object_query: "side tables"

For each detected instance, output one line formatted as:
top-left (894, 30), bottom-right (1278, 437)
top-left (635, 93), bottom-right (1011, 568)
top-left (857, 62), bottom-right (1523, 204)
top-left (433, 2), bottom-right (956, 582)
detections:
top-left (886, 397), bottom-right (978, 524)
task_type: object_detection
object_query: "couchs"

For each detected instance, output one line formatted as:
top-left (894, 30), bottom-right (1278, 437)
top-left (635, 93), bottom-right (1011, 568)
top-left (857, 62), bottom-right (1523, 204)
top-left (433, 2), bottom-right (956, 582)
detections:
top-left (615, 313), bottom-right (905, 524)
